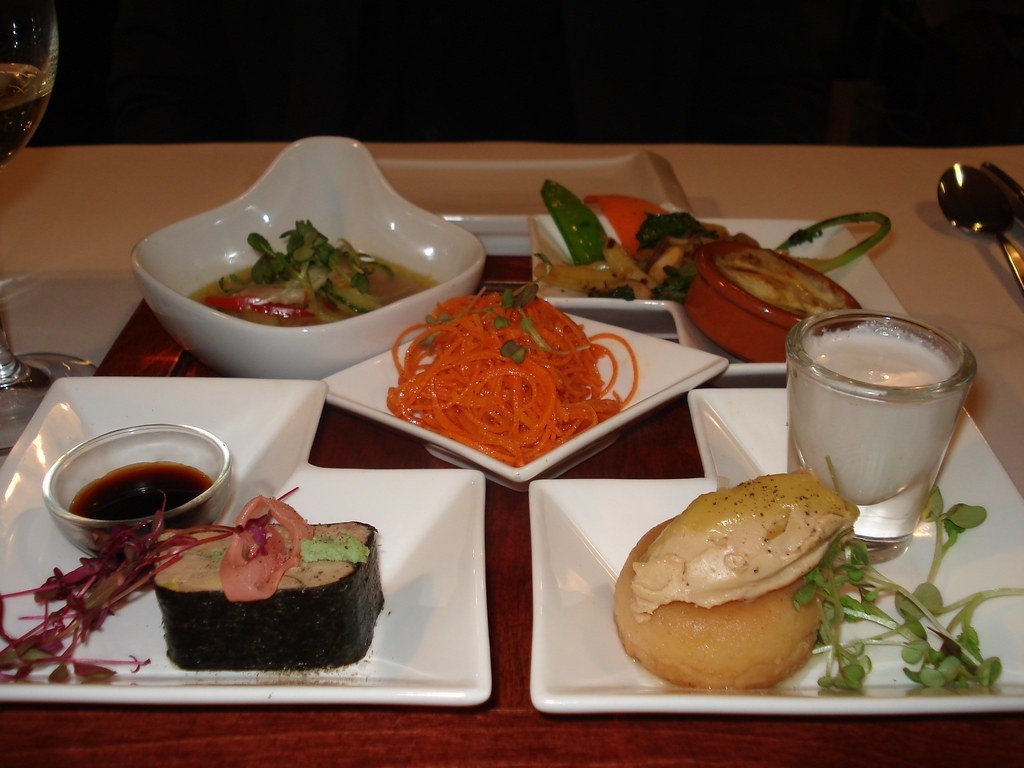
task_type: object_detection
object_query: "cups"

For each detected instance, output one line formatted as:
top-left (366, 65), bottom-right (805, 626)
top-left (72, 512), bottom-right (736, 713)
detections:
top-left (785, 305), bottom-right (978, 563)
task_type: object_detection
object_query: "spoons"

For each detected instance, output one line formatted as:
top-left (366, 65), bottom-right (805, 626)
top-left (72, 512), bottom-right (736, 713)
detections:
top-left (936, 163), bottom-right (1024, 297)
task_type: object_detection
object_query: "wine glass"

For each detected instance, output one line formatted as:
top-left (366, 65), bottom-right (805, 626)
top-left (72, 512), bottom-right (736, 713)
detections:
top-left (0, 1), bottom-right (101, 455)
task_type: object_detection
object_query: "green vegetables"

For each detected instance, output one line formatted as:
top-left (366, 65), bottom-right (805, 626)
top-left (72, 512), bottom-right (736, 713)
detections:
top-left (589, 210), bottom-right (892, 303)
top-left (220, 219), bottom-right (393, 313)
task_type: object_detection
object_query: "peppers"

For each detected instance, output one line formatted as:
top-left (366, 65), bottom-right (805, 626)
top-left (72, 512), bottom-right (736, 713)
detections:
top-left (584, 192), bottom-right (668, 257)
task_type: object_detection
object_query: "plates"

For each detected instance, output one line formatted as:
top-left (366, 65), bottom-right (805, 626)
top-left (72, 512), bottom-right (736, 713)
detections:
top-left (529, 213), bottom-right (916, 379)
top-left (525, 384), bottom-right (1024, 720)
top-left (1, 377), bottom-right (491, 705)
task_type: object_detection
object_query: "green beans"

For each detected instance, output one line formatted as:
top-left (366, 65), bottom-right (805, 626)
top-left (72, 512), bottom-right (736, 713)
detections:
top-left (540, 178), bottom-right (609, 264)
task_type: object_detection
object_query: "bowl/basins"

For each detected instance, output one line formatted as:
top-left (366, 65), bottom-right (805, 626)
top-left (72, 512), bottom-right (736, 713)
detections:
top-left (41, 424), bottom-right (233, 562)
top-left (685, 243), bottom-right (864, 364)
top-left (128, 135), bottom-right (488, 381)
top-left (317, 312), bottom-right (727, 493)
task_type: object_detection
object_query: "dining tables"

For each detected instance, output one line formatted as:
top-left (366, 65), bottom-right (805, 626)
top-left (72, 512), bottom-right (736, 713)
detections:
top-left (0, 142), bottom-right (1024, 768)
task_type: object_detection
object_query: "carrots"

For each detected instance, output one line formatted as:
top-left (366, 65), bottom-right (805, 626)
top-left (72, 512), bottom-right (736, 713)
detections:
top-left (387, 290), bottom-right (638, 467)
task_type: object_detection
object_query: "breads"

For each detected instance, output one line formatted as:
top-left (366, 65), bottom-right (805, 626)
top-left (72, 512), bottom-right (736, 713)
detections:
top-left (612, 512), bottom-right (822, 690)
